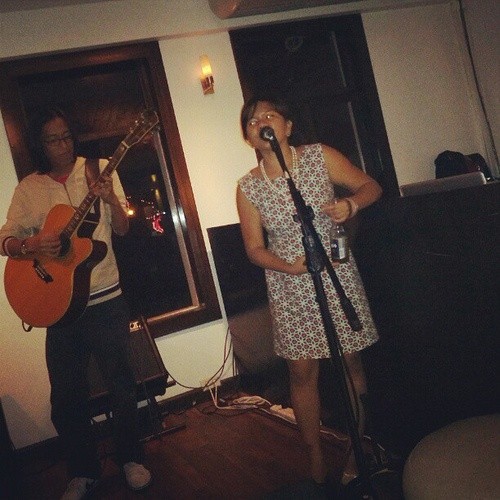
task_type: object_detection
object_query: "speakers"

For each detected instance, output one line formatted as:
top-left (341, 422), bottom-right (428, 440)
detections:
top-left (83, 315), bottom-right (168, 416)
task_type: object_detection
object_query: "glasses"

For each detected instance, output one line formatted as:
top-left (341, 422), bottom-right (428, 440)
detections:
top-left (42, 131), bottom-right (73, 146)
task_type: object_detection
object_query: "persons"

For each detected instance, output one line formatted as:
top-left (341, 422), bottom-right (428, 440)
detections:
top-left (0, 104), bottom-right (154, 500)
top-left (236, 93), bottom-right (384, 500)
top-left (403, 415), bottom-right (500, 500)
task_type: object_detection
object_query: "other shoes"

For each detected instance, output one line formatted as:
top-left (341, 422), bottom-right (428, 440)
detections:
top-left (309, 454), bottom-right (326, 482)
top-left (342, 449), bottom-right (359, 485)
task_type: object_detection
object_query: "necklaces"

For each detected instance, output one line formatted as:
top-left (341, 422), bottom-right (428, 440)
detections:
top-left (260, 146), bottom-right (300, 198)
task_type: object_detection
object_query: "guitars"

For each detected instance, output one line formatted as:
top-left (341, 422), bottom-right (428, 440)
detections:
top-left (4, 107), bottom-right (159, 330)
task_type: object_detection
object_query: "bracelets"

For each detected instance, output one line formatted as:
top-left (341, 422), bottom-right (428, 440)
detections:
top-left (343, 197), bottom-right (358, 219)
top-left (19, 238), bottom-right (28, 256)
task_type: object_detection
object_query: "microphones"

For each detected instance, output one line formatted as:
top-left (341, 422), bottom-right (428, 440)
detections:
top-left (259, 126), bottom-right (276, 143)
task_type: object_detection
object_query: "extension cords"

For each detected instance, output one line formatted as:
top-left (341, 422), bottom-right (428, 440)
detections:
top-left (270, 404), bottom-right (323, 426)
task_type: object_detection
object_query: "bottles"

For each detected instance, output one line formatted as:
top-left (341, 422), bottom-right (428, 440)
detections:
top-left (329, 197), bottom-right (349, 264)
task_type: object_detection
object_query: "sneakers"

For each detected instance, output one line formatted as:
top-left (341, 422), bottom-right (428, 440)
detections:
top-left (123, 461), bottom-right (153, 491)
top-left (61, 477), bottom-right (95, 500)
